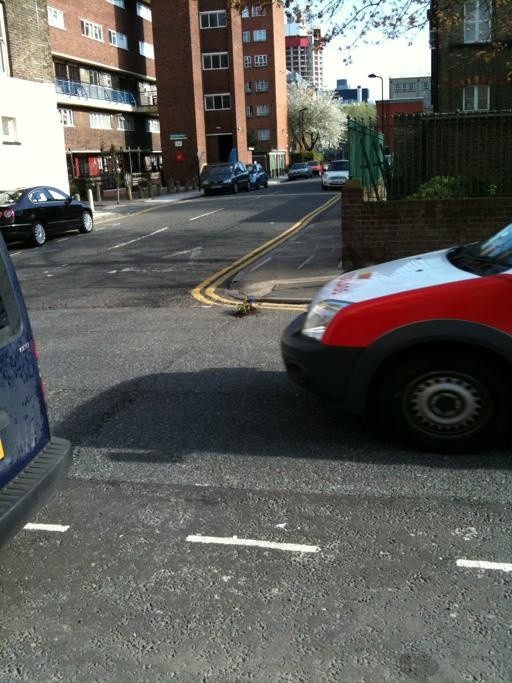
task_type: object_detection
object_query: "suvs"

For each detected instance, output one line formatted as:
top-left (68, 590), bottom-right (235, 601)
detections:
top-left (201, 161), bottom-right (251, 193)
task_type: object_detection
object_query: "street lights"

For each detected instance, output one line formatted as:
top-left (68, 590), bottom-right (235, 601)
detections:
top-left (368, 73), bottom-right (385, 134)
top-left (299, 107), bottom-right (308, 161)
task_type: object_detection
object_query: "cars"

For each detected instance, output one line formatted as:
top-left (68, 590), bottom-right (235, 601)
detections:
top-left (245, 163), bottom-right (269, 189)
top-left (308, 160), bottom-right (323, 175)
top-left (322, 159), bottom-right (350, 190)
top-left (279, 221), bottom-right (510, 454)
top-left (0, 184), bottom-right (96, 250)
top-left (288, 162), bottom-right (314, 178)
top-left (1, 224), bottom-right (75, 549)
